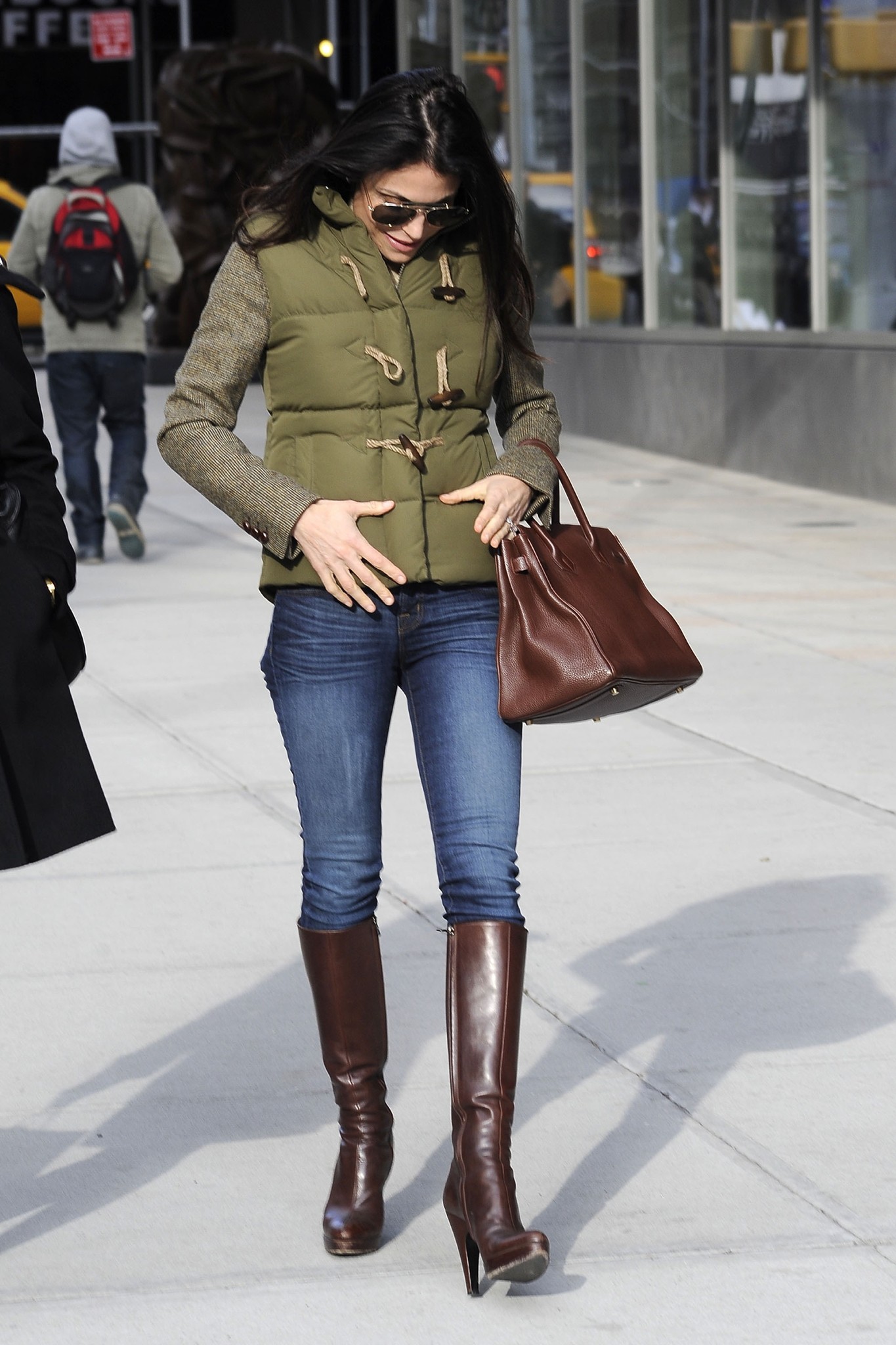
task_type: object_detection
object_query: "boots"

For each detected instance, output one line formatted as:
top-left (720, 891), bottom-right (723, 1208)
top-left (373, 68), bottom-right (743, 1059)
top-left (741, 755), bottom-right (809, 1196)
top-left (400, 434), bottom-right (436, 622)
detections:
top-left (297, 915), bottom-right (393, 1255)
top-left (437, 920), bottom-right (550, 1297)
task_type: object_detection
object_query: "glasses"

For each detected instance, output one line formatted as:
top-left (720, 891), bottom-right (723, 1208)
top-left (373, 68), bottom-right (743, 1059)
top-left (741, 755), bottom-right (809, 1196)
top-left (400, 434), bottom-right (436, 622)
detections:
top-left (359, 172), bottom-right (469, 227)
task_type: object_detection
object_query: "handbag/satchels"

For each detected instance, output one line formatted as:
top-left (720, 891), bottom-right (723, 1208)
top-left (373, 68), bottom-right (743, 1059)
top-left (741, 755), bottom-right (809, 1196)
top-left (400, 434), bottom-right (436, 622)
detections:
top-left (495, 439), bottom-right (703, 724)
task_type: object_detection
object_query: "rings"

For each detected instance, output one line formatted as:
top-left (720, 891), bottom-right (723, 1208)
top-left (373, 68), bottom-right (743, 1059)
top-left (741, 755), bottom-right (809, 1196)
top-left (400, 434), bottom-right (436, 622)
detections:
top-left (505, 517), bottom-right (514, 532)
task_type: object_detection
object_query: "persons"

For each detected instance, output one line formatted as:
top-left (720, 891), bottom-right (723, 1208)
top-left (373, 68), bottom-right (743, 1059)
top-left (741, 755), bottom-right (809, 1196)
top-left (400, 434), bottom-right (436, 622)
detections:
top-left (0, 254), bottom-right (116, 874)
top-left (156, 68), bottom-right (703, 1294)
top-left (7, 108), bottom-right (183, 567)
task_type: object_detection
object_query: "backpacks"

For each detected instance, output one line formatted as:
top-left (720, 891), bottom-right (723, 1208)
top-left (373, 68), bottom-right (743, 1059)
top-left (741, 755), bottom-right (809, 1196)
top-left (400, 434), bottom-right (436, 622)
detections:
top-left (40, 173), bottom-right (138, 329)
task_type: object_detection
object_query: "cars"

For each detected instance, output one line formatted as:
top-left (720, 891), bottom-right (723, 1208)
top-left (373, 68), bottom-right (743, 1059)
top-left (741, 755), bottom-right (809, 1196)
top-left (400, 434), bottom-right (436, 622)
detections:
top-left (0, 177), bottom-right (47, 368)
top-left (498, 166), bottom-right (642, 324)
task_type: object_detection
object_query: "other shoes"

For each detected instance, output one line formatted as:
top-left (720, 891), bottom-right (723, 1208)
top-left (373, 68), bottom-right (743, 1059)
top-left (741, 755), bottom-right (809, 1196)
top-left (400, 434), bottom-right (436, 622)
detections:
top-left (75, 544), bottom-right (104, 566)
top-left (106, 494), bottom-right (145, 560)
